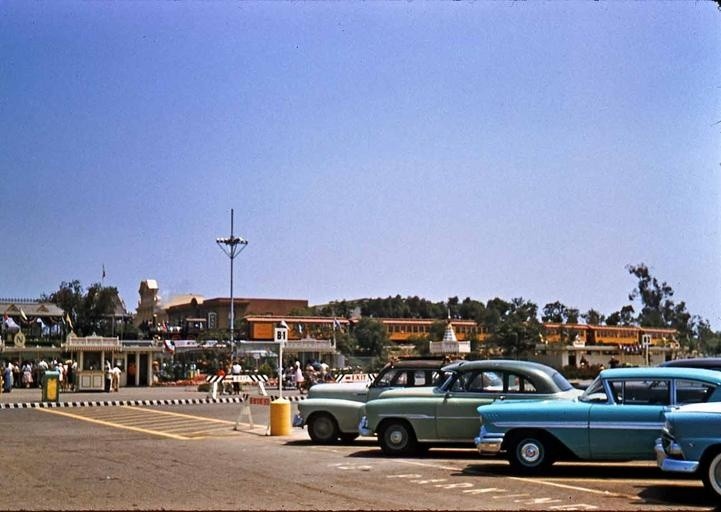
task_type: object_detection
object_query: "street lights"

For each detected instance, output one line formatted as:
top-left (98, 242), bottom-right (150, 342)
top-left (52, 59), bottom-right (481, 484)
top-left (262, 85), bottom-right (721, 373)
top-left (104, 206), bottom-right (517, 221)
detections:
top-left (218, 208), bottom-right (251, 376)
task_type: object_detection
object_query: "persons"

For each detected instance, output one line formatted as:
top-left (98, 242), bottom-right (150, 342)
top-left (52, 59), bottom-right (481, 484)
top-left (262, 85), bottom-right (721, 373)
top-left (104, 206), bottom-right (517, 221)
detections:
top-left (320, 360), bottom-right (329, 381)
top-left (231, 362), bottom-right (242, 394)
top-left (608, 356), bottom-right (619, 368)
top-left (112, 365), bottom-right (122, 392)
top-left (1, 358), bottom-right (79, 393)
top-left (384, 356), bottom-right (407, 384)
top-left (292, 356), bottom-right (305, 394)
top-left (342, 359), bottom-right (352, 370)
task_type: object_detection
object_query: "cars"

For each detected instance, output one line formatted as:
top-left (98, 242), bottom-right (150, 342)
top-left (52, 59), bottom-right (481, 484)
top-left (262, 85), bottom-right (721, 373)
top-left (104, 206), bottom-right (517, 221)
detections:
top-left (292, 361), bottom-right (502, 445)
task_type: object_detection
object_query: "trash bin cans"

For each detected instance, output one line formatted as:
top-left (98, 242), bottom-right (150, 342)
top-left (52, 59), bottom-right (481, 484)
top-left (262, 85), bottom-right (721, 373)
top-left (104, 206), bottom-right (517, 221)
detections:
top-left (187, 370), bottom-right (197, 380)
top-left (42, 371), bottom-right (59, 402)
